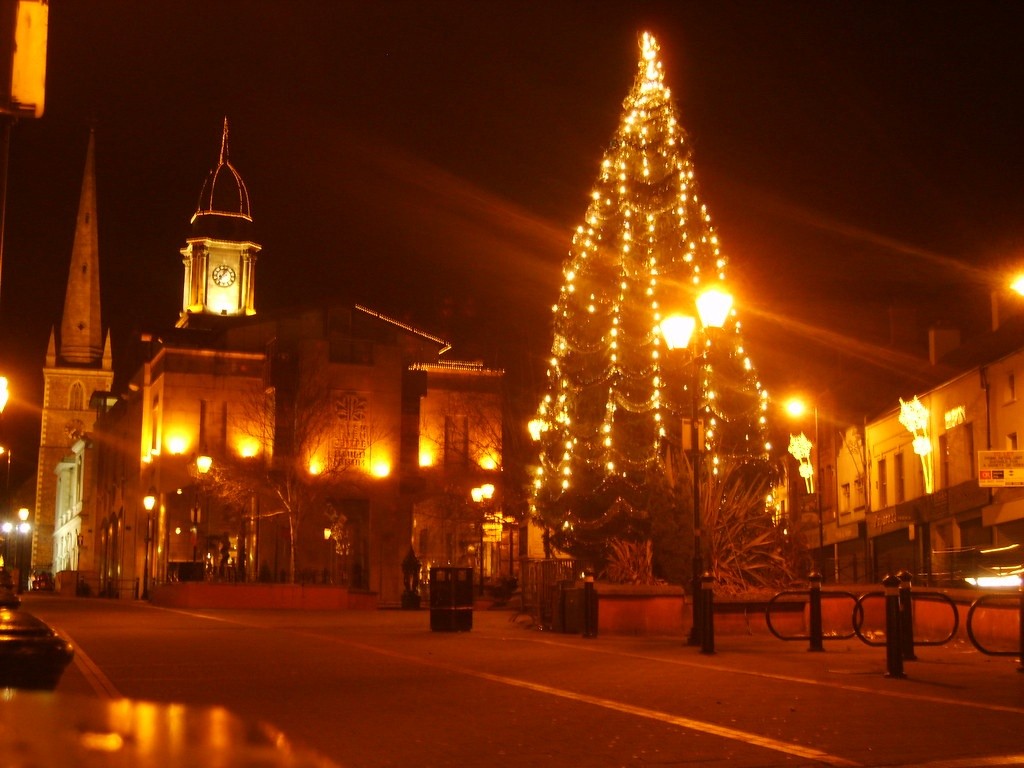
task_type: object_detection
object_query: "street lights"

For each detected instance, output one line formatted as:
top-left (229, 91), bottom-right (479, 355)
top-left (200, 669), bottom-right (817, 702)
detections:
top-left (16, 508), bottom-right (29, 594)
top-left (192, 453), bottom-right (213, 563)
top-left (659, 282), bottom-right (733, 649)
top-left (470, 482), bottom-right (495, 595)
top-left (143, 489), bottom-right (156, 599)
top-left (785, 396), bottom-right (825, 581)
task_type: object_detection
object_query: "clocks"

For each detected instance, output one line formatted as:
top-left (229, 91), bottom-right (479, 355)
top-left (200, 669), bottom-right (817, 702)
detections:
top-left (212, 265), bottom-right (236, 287)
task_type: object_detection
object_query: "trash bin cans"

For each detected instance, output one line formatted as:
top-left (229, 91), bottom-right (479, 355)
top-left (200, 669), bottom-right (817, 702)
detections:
top-left (429, 566), bottom-right (473, 634)
